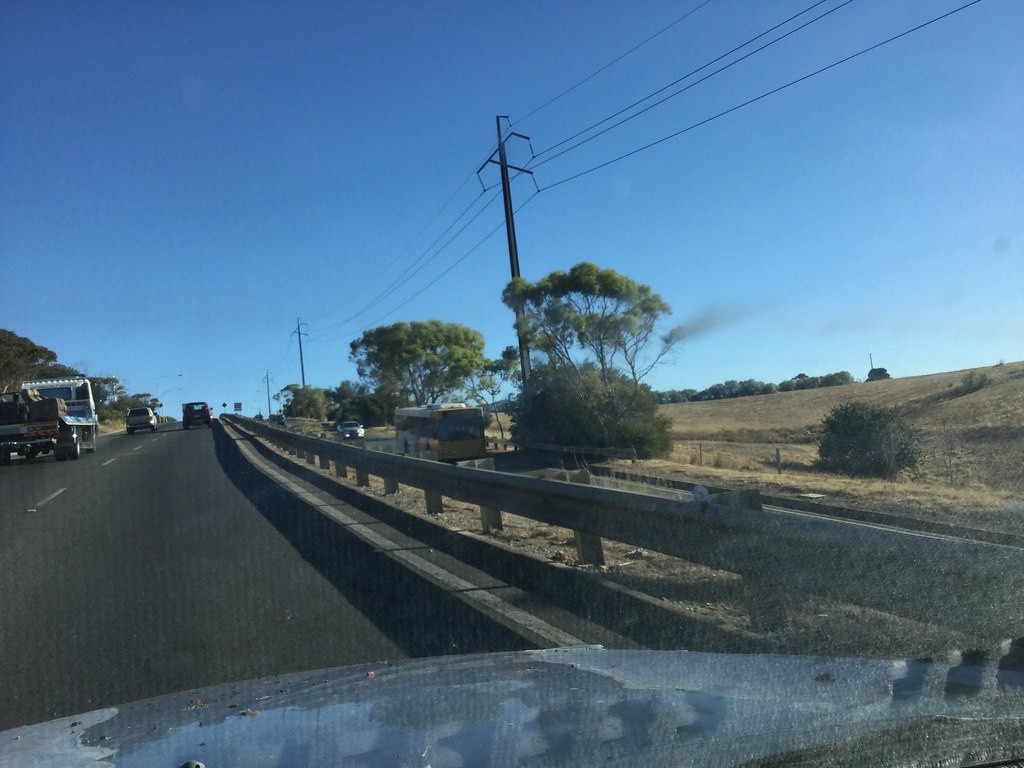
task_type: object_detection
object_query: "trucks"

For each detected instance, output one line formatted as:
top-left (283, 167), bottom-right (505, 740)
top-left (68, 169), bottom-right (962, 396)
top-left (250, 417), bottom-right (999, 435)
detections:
top-left (1, 373), bottom-right (100, 466)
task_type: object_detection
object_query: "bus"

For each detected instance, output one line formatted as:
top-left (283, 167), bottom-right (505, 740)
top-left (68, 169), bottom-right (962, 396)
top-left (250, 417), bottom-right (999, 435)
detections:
top-left (394, 403), bottom-right (487, 463)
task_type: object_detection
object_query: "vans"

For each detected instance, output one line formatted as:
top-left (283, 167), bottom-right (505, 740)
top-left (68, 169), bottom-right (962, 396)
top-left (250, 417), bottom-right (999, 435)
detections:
top-left (269, 413), bottom-right (286, 425)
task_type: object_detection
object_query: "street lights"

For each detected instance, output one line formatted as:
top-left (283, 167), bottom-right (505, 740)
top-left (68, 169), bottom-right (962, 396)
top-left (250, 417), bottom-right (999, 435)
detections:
top-left (156, 374), bottom-right (182, 423)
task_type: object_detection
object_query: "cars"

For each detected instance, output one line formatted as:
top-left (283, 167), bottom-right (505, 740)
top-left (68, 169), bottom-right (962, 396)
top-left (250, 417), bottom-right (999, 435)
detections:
top-left (256, 414), bottom-right (264, 420)
top-left (337, 421), bottom-right (365, 439)
top-left (183, 402), bottom-right (213, 428)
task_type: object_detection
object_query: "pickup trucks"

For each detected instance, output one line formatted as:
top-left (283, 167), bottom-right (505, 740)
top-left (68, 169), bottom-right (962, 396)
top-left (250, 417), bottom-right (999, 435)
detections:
top-left (126, 407), bottom-right (157, 433)
